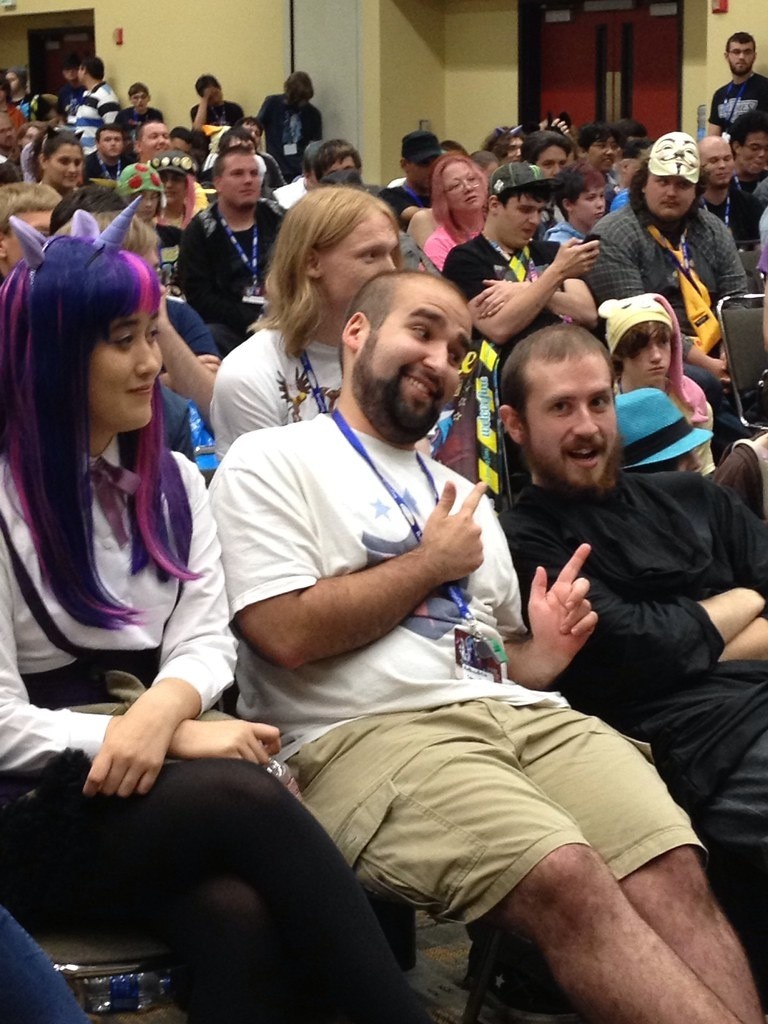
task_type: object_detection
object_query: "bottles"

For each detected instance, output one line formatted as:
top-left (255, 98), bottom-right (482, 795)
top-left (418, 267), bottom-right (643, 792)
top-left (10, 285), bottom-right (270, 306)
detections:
top-left (80, 974), bottom-right (172, 1014)
top-left (264, 757), bottom-right (305, 808)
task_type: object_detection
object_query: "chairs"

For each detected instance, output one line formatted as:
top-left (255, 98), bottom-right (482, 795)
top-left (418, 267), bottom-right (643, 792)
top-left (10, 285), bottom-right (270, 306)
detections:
top-left (717, 294), bottom-right (768, 433)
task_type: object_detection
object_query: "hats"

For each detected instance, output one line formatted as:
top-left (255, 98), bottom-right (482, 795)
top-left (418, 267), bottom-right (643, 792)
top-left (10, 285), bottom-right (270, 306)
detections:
top-left (147, 150), bottom-right (198, 227)
top-left (598, 293), bottom-right (709, 423)
top-left (7, 67), bottom-right (28, 86)
top-left (614, 387), bottom-right (714, 469)
top-left (489, 162), bottom-right (557, 195)
top-left (401, 130), bottom-right (441, 163)
top-left (322, 169), bottom-right (379, 196)
top-left (119, 162), bottom-right (167, 208)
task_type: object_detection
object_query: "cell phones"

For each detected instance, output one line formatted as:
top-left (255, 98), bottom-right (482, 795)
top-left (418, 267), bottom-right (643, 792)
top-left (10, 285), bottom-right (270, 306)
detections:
top-left (580, 234), bottom-right (600, 253)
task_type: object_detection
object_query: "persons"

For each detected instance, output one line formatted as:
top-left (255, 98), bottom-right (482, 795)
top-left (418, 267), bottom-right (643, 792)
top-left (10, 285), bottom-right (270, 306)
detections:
top-left (0, 32), bottom-right (768, 1024)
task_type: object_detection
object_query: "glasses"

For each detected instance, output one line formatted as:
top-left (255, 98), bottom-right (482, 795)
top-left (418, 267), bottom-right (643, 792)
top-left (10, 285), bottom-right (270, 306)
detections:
top-left (745, 143), bottom-right (768, 151)
top-left (728, 49), bottom-right (752, 54)
top-left (445, 174), bottom-right (479, 193)
